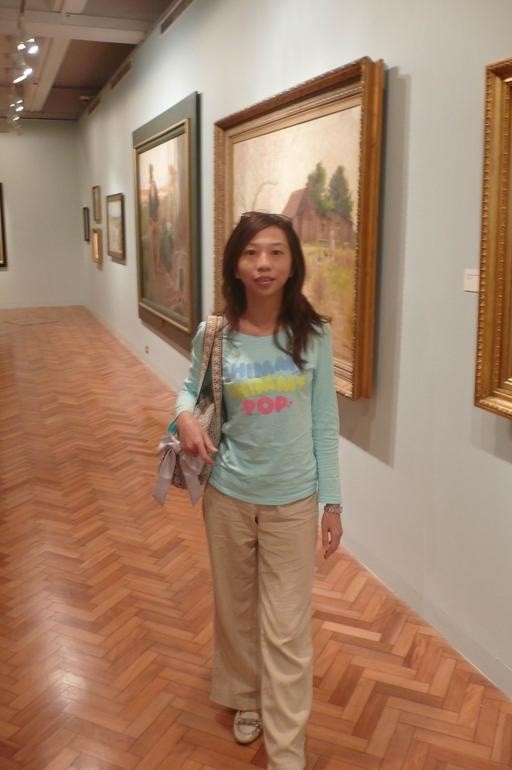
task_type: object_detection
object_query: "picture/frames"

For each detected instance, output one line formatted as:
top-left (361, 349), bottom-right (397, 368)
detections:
top-left (209, 55), bottom-right (387, 400)
top-left (129, 90), bottom-right (202, 337)
top-left (81, 184), bottom-right (124, 264)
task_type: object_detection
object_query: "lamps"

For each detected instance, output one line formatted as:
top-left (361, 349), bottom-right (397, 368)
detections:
top-left (6, 16), bottom-right (38, 131)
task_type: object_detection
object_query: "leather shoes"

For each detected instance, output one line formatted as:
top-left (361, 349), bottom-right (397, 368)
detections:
top-left (232, 709), bottom-right (263, 745)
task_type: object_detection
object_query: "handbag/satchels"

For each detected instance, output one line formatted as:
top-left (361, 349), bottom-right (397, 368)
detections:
top-left (156, 314), bottom-right (224, 499)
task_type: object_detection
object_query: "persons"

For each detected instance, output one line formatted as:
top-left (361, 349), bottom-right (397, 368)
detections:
top-left (166, 165), bottom-right (180, 281)
top-left (168, 210), bottom-right (343, 770)
top-left (146, 164), bottom-right (163, 278)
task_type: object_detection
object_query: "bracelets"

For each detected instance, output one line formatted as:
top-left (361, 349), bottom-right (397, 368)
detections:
top-left (324, 504), bottom-right (343, 514)
